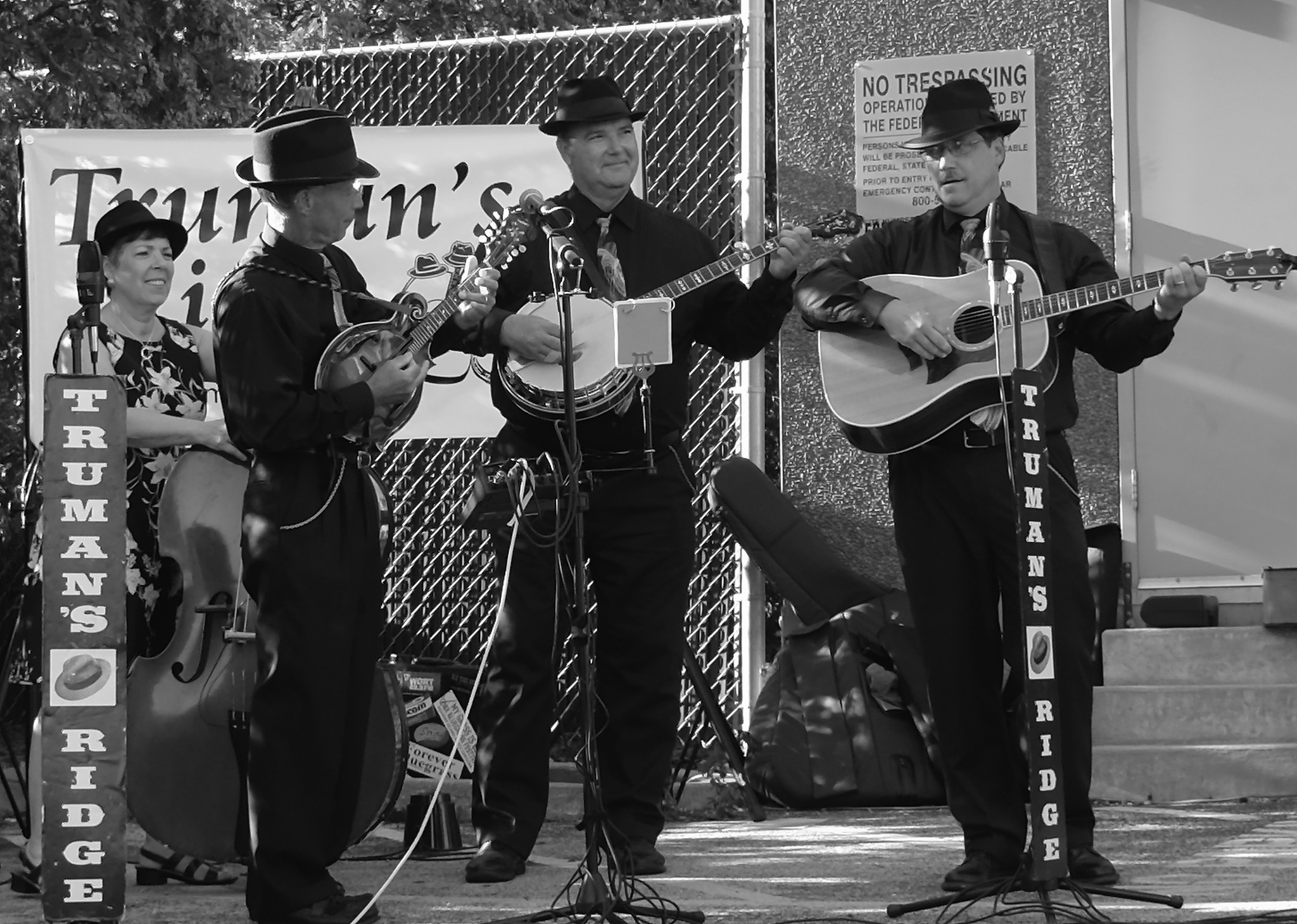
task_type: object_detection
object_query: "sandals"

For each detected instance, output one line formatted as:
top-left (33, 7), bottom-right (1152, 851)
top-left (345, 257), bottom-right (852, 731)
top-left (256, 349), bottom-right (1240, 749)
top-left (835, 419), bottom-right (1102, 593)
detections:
top-left (9, 841), bottom-right (42, 893)
top-left (134, 846), bottom-right (239, 886)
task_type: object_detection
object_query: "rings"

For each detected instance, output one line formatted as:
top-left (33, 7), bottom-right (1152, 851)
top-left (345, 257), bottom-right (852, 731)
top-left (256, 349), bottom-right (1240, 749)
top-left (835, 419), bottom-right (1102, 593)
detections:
top-left (1175, 281), bottom-right (1184, 285)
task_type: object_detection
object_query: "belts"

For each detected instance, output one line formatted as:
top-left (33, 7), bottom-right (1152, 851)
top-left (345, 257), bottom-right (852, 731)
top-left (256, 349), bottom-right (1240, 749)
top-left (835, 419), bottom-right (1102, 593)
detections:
top-left (309, 440), bottom-right (383, 470)
top-left (581, 428), bottom-right (682, 467)
top-left (922, 424), bottom-right (1066, 451)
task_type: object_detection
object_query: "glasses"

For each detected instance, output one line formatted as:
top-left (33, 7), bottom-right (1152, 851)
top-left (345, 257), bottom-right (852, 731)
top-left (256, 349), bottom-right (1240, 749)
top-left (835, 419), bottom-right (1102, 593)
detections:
top-left (918, 134), bottom-right (990, 161)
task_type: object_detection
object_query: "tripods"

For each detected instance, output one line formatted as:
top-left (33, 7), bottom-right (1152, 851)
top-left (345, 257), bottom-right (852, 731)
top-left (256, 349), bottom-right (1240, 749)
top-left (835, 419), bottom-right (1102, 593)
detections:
top-left (886, 265), bottom-right (1184, 924)
top-left (484, 211), bottom-right (707, 924)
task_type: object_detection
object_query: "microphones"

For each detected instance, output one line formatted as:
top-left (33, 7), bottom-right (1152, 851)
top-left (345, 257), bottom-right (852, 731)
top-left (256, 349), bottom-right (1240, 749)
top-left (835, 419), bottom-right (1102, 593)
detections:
top-left (520, 189), bottom-right (550, 215)
top-left (75, 240), bottom-right (105, 364)
top-left (982, 201), bottom-right (1009, 317)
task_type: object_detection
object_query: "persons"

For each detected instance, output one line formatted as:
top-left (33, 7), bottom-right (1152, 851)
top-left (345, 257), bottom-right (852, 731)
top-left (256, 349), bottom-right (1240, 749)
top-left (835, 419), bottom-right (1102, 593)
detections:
top-left (217, 110), bottom-right (500, 924)
top-left (10, 200), bottom-right (247, 894)
top-left (795, 78), bottom-right (1208, 890)
top-left (451, 75), bottom-right (812, 883)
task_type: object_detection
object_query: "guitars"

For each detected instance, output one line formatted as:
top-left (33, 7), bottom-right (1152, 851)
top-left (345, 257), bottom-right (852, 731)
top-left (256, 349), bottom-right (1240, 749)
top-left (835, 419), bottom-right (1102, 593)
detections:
top-left (497, 210), bottom-right (865, 420)
top-left (818, 245), bottom-right (1297, 454)
top-left (126, 86), bottom-right (408, 861)
top-left (313, 207), bottom-right (531, 444)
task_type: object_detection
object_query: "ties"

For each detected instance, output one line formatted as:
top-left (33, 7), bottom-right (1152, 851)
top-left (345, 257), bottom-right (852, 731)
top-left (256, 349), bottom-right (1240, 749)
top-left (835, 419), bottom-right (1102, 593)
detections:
top-left (312, 253), bottom-right (359, 336)
top-left (957, 216), bottom-right (1005, 431)
top-left (593, 213), bottom-right (636, 417)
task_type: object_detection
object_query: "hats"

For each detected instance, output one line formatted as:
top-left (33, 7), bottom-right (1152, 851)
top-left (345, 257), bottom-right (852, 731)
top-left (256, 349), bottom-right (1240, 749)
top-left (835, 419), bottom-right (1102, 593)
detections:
top-left (233, 108), bottom-right (383, 187)
top-left (893, 77), bottom-right (1024, 157)
top-left (92, 199), bottom-right (191, 263)
top-left (534, 77), bottom-right (652, 135)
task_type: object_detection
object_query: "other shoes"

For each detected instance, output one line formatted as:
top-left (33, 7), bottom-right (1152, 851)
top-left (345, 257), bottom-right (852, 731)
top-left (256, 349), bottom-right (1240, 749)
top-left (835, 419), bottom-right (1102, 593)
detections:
top-left (941, 849), bottom-right (1014, 892)
top-left (606, 838), bottom-right (666, 875)
top-left (1069, 845), bottom-right (1119, 888)
top-left (264, 892), bottom-right (380, 924)
top-left (465, 841), bottom-right (526, 883)
